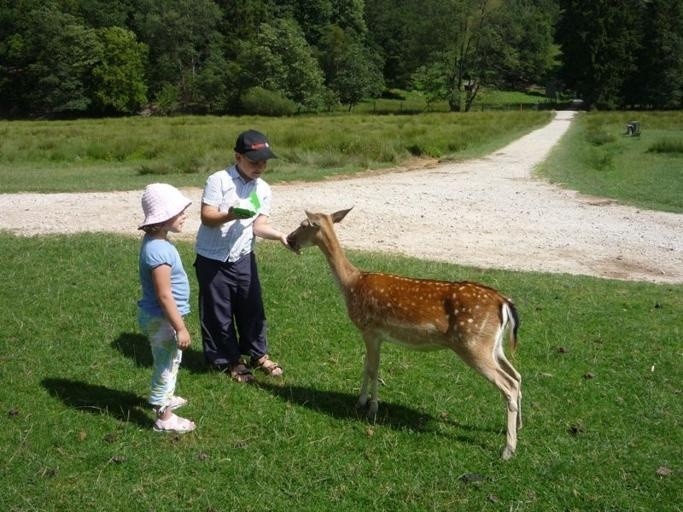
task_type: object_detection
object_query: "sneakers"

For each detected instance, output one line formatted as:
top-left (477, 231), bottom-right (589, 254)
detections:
top-left (151, 415), bottom-right (198, 434)
top-left (151, 394), bottom-right (189, 413)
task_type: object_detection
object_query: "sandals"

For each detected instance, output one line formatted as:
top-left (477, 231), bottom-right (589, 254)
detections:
top-left (251, 354), bottom-right (285, 378)
top-left (218, 357), bottom-right (257, 384)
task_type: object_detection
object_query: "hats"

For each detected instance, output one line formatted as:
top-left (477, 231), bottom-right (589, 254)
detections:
top-left (234, 128), bottom-right (278, 163)
top-left (137, 183), bottom-right (193, 230)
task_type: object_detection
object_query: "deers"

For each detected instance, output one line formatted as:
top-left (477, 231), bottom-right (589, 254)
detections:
top-left (284, 205), bottom-right (524, 463)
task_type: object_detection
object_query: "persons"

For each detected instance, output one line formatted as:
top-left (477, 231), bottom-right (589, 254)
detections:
top-left (135, 182), bottom-right (196, 432)
top-left (196, 128), bottom-right (303, 384)
